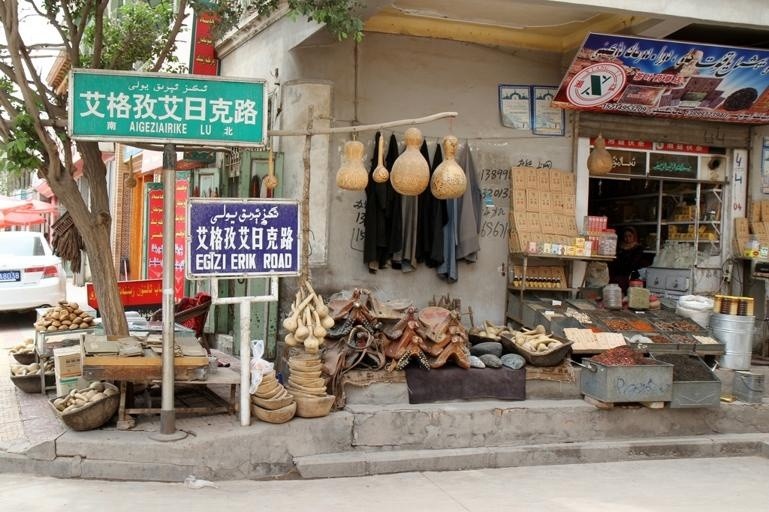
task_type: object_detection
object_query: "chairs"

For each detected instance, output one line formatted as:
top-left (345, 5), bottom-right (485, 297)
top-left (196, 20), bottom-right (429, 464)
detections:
top-left (149, 291), bottom-right (213, 353)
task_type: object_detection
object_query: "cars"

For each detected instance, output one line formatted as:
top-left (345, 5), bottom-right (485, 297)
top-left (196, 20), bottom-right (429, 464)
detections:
top-left (0, 229), bottom-right (72, 317)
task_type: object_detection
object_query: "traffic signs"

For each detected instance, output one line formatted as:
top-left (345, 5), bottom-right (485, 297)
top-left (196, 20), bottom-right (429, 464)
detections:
top-left (68, 67), bottom-right (268, 150)
top-left (186, 197), bottom-right (302, 278)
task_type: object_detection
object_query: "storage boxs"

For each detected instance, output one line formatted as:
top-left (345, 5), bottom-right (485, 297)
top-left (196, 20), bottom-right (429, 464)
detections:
top-left (499, 289), bottom-right (728, 408)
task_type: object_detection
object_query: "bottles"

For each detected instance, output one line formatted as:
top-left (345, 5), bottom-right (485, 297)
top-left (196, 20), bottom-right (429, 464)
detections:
top-left (513, 276), bottom-right (561, 289)
top-left (627, 280), bottom-right (650, 310)
top-left (649, 296), bottom-right (660, 309)
top-left (598, 227), bottom-right (619, 254)
top-left (603, 282), bottom-right (622, 309)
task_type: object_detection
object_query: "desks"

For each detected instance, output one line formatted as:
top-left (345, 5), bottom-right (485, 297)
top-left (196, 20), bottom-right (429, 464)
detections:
top-left (115, 346), bottom-right (241, 424)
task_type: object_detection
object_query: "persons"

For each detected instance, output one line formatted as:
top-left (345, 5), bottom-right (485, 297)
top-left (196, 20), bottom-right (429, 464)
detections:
top-left (609, 226), bottom-right (645, 296)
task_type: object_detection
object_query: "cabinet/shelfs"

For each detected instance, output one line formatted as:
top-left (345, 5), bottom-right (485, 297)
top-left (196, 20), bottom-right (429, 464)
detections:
top-left (659, 178), bottom-right (726, 272)
top-left (507, 251), bottom-right (616, 302)
top-left (34, 324), bottom-right (103, 399)
top-left (641, 267), bottom-right (725, 308)
top-left (617, 188), bottom-right (658, 267)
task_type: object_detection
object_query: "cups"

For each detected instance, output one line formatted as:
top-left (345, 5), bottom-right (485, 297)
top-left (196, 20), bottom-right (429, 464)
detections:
top-left (527, 240), bottom-right (536, 252)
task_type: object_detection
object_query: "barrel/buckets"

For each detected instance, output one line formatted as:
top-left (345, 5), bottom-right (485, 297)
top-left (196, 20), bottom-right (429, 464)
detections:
top-left (708, 314), bottom-right (756, 370)
top-left (728, 373), bottom-right (765, 407)
